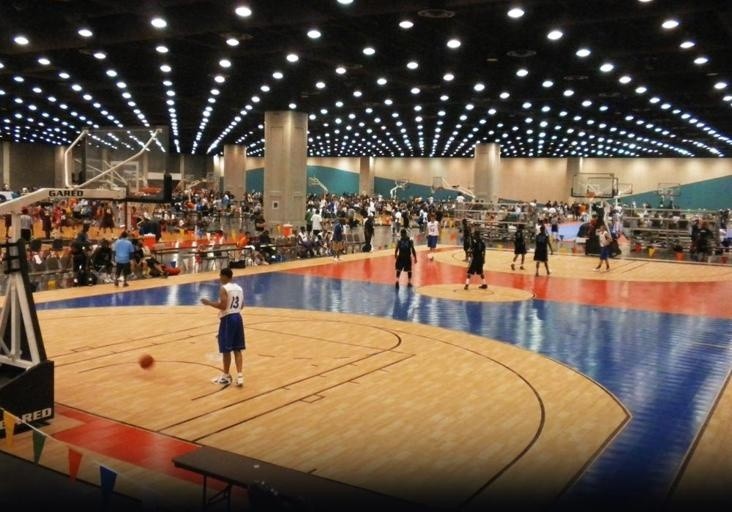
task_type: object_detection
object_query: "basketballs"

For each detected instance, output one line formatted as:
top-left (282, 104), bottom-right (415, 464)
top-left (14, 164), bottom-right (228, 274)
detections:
top-left (579, 205), bottom-right (586, 213)
top-left (139, 354), bottom-right (154, 369)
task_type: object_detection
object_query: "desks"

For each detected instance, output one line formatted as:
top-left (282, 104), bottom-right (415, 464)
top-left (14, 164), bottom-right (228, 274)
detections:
top-left (172, 444), bottom-right (428, 511)
top-left (190, 242), bottom-right (296, 265)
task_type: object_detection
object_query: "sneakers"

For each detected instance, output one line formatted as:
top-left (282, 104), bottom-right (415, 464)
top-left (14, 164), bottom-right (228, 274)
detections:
top-left (465, 284), bottom-right (468, 289)
top-left (511, 264), bottom-right (524, 270)
top-left (479, 285), bottom-right (485, 288)
top-left (235, 374), bottom-right (243, 385)
top-left (212, 375), bottom-right (231, 384)
top-left (536, 271), bottom-right (552, 276)
top-left (396, 280), bottom-right (411, 288)
top-left (593, 267), bottom-right (610, 272)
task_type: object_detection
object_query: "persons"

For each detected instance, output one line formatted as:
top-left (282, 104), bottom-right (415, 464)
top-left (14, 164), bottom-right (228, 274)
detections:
top-left (201, 268), bottom-right (245, 386)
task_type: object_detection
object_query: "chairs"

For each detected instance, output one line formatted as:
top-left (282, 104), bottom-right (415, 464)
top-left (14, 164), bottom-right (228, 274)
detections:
top-left (25, 255), bottom-right (74, 289)
top-left (341, 234), bottom-right (365, 254)
top-left (148, 236), bottom-right (240, 272)
